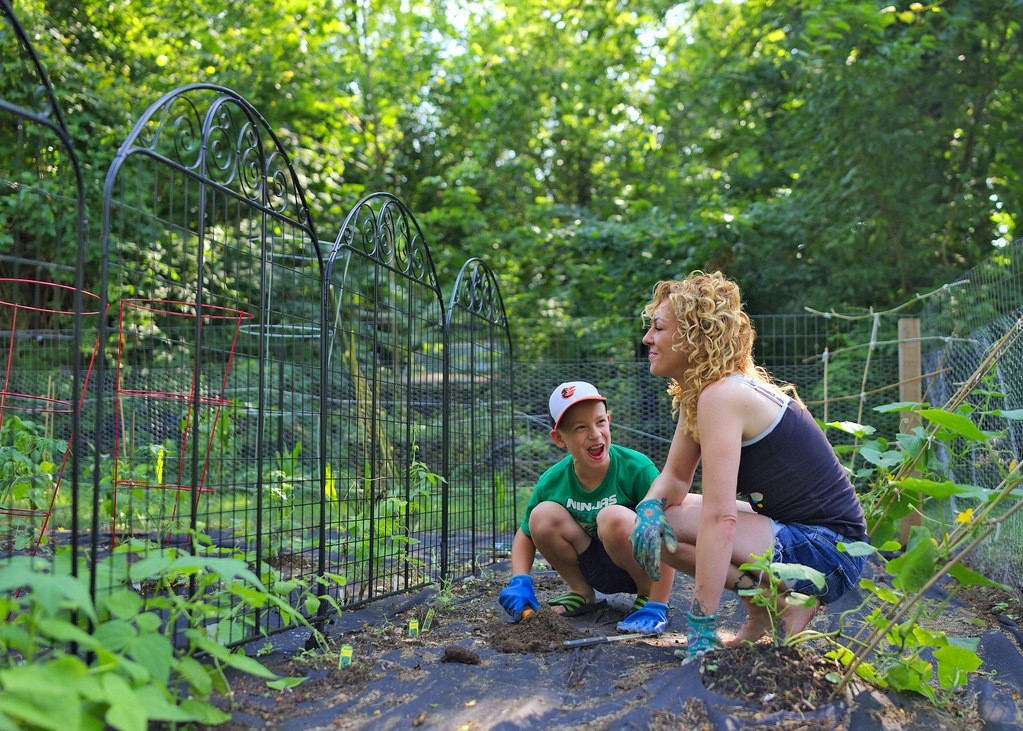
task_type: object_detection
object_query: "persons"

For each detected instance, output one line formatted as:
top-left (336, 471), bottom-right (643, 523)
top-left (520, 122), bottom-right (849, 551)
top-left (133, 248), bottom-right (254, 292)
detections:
top-left (498, 380), bottom-right (676, 635)
top-left (628, 269), bottom-right (869, 667)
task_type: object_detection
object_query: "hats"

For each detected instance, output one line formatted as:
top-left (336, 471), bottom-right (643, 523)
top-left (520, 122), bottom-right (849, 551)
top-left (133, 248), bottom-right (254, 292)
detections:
top-left (547, 380), bottom-right (607, 431)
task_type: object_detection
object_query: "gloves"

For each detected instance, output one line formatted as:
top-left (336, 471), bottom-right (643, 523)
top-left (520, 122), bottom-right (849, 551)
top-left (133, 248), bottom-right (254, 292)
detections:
top-left (628, 496), bottom-right (677, 582)
top-left (616, 600), bottom-right (670, 635)
top-left (674, 610), bottom-right (728, 666)
top-left (498, 572), bottom-right (540, 622)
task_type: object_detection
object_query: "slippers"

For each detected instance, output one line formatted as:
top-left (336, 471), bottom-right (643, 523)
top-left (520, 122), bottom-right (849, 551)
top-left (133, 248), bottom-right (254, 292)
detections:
top-left (546, 591), bottom-right (608, 617)
top-left (623, 595), bottom-right (669, 619)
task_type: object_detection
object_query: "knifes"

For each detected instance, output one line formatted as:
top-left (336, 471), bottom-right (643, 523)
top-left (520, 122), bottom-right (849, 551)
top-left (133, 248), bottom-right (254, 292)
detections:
top-left (562, 633), bottom-right (658, 649)
